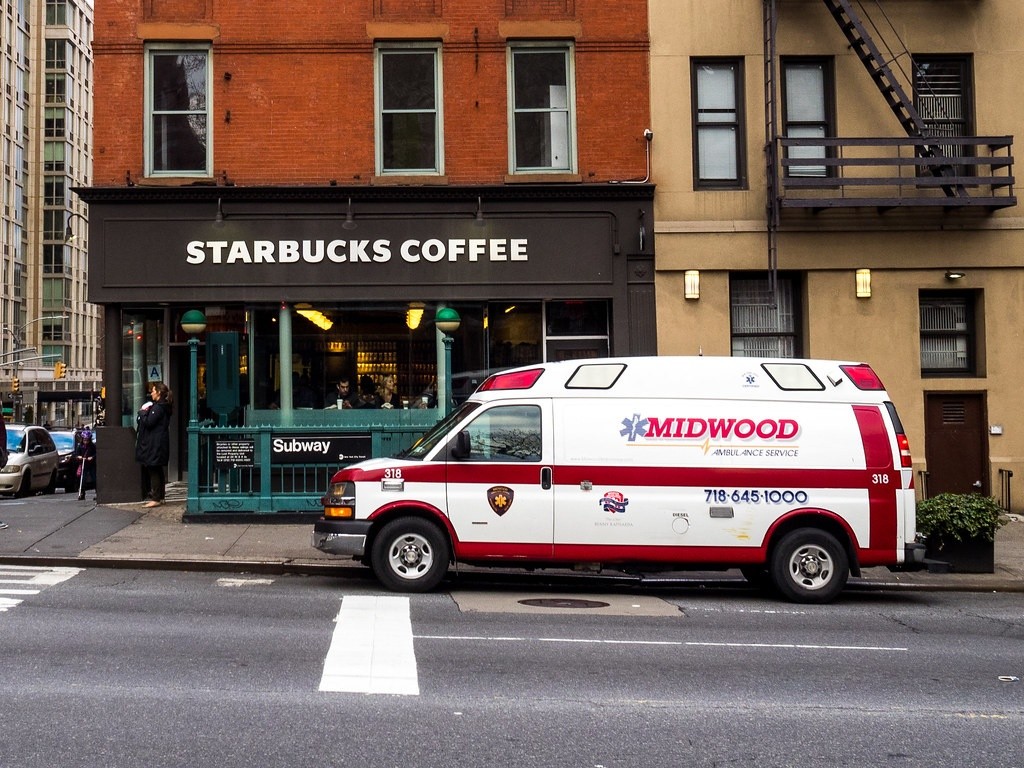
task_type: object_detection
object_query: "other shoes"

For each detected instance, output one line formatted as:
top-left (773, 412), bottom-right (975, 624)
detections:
top-left (0, 521), bottom-right (9, 529)
top-left (141, 500), bottom-right (165, 508)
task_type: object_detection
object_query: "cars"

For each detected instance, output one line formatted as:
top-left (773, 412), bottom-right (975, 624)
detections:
top-left (0, 421), bottom-right (59, 499)
top-left (50, 429), bottom-right (76, 482)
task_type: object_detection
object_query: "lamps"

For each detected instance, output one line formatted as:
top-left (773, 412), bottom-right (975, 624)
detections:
top-left (341, 197), bottom-right (358, 231)
top-left (944, 268), bottom-right (966, 281)
top-left (472, 195), bottom-right (487, 227)
top-left (64, 213), bottom-right (88, 243)
top-left (212, 197), bottom-right (227, 231)
top-left (855, 268), bottom-right (872, 299)
top-left (684, 270), bottom-right (700, 300)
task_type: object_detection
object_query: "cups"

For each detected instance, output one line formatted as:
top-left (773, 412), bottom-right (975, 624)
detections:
top-left (337, 399), bottom-right (343, 410)
top-left (422, 397), bottom-right (428, 409)
top-left (403, 401), bottom-right (409, 409)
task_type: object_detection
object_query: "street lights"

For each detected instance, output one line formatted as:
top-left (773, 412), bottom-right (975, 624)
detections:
top-left (3, 314), bottom-right (70, 379)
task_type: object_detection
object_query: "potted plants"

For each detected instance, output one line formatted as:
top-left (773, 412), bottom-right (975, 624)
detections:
top-left (916, 492), bottom-right (1011, 575)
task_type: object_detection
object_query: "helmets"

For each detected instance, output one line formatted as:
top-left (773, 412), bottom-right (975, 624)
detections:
top-left (81, 430), bottom-right (92, 438)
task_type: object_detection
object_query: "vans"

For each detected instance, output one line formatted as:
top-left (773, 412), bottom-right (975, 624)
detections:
top-left (313, 354), bottom-right (917, 605)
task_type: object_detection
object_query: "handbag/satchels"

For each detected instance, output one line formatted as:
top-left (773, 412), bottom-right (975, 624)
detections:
top-left (77, 465), bottom-right (83, 478)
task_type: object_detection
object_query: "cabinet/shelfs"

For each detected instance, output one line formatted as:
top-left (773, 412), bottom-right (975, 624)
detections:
top-left (356, 339), bottom-right (399, 386)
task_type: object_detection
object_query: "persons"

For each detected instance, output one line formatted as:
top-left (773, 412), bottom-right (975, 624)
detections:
top-left (72, 424), bottom-right (96, 495)
top-left (324, 377), bottom-right (359, 409)
top-left (134, 382), bottom-right (173, 508)
top-left (355, 376), bottom-right (384, 409)
top-left (0, 412), bottom-right (9, 530)
top-left (43, 421), bottom-right (52, 430)
top-left (265, 372), bottom-right (317, 410)
top-left (378, 374), bottom-right (400, 409)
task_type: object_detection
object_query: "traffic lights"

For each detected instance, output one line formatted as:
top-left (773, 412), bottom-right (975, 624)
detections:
top-left (11, 376), bottom-right (19, 394)
top-left (54, 362), bottom-right (67, 380)
